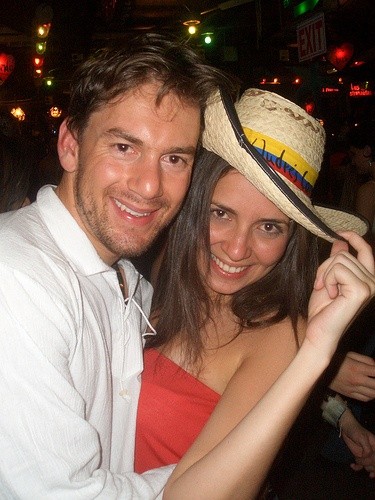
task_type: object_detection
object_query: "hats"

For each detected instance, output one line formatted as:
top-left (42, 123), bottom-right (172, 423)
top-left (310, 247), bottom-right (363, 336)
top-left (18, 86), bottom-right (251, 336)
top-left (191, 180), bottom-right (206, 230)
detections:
top-left (199, 85), bottom-right (369, 243)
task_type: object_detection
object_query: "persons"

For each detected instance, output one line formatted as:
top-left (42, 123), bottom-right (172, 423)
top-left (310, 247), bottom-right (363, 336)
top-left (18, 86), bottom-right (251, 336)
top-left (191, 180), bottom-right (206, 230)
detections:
top-left (0, 31), bottom-right (227, 500)
top-left (134, 81), bottom-right (375, 500)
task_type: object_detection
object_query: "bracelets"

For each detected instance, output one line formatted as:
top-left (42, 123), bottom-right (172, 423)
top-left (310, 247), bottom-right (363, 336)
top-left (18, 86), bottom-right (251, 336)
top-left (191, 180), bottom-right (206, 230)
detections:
top-left (322, 392), bottom-right (347, 432)
